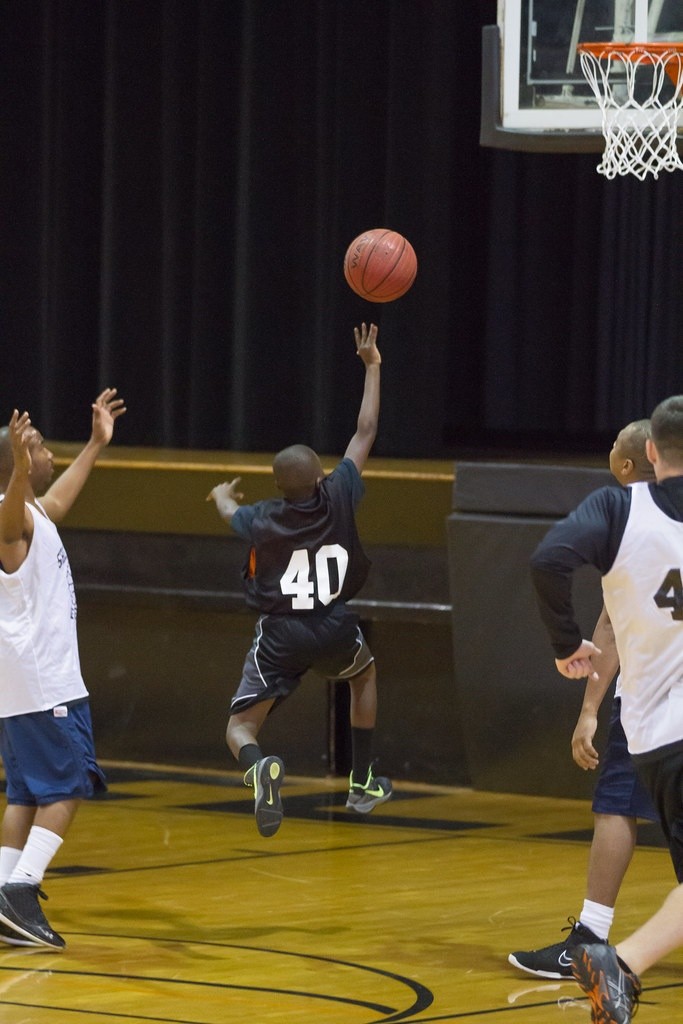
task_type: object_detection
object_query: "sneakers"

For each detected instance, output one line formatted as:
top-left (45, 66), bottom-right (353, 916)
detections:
top-left (243, 755), bottom-right (287, 838)
top-left (509, 915), bottom-right (608, 980)
top-left (0, 883), bottom-right (66, 949)
top-left (345, 762), bottom-right (392, 813)
top-left (572, 942), bottom-right (642, 1024)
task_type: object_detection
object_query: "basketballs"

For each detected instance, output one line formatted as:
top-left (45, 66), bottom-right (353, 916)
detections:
top-left (343, 228), bottom-right (418, 303)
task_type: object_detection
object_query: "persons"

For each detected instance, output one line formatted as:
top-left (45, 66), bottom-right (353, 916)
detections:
top-left (1, 388), bottom-right (127, 951)
top-left (508, 420), bottom-right (662, 979)
top-left (531, 395), bottom-right (683, 1024)
top-left (206, 321), bottom-right (395, 838)
